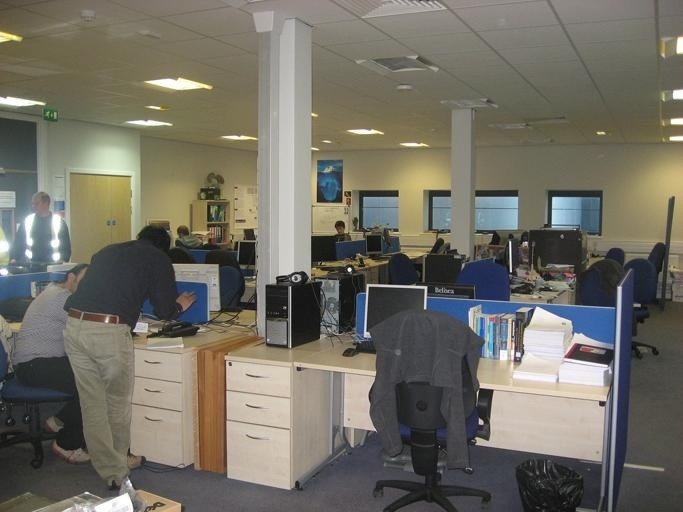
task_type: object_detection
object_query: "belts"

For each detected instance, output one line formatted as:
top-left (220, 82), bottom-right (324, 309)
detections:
top-left (68, 309), bottom-right (126, 324)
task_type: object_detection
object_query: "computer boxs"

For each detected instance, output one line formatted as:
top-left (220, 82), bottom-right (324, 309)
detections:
top-left (314, 273), bottom-right (366, 335)
top-left (265, 282), bottom-right (322, 349)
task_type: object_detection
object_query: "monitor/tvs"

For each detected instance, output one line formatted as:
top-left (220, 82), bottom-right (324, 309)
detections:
top-left (362, 284), bottom-right (428, 341)
top-left (529, 228), bottom-right (581, 273)
top-left (311, 235), bottom-right (337, 262)
top-left (237, 240), bottom-right (256, 281)
top-left (416, 282), bottom-right (477, 299)
top-left (508, 239), bottom-right (519, 276)
top-left (366, 235), bottom-right (383, 259)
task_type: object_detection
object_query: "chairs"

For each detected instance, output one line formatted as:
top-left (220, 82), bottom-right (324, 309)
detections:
top-left (646, 242), bottom-right (665, 273)
top-left (0, 314), bottom-right (74, 469)
top-left (575, 259), bottom-right (625, 306)
top-left (604, 247), bottom-right (625, 264)
top-left (169, 246), bottom-right (196, 263)
top-left (388, 253), bottom-right (418, 286)
top-left (206, 248), bottom-right (238, 266)
top-left (626, 258), bottom-right (660, 359)
top-left (220, 266), bottom-right (245, 310)
top-left (368, 311), bottom-right (491, 511)
top-left (452, 257), bottom-right (510, 301)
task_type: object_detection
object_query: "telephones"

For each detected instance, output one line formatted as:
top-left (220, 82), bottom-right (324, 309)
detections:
top-left (147, 321), bottom-right (199, 338)
top-left (511, 284), bottom-right (533, 294)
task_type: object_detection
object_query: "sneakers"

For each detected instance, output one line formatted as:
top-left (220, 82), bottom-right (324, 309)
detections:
top-left (43, 416), bottom-right (63, 433)
top-left (127, 457), bottom-right (146, 468)
top-left (51, 439), bottom-right (90, 463)
top-left (107, 477), bottom-right (121, 488)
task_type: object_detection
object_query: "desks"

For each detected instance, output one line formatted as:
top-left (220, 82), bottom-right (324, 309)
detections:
top-left (294, 321), bottom-right (614, 509)
top-left (509, 262), bottom-right (574, 304)
top-left (311, 249), bottom-right (426, 280)
top-left (131, 298), bottom-right (260, 349)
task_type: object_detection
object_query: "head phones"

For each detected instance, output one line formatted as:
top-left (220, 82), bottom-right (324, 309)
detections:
top-left (339, 265), bottom-right (356, 275)
top-left (276, 271), bottom-right (309, 284)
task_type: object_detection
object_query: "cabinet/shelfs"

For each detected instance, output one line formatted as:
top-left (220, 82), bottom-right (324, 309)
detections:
top-left (225, 357), bottom-right (292, 493)
top-left (191, 199), bottom-right (230, 242)
top-left (128, 342), bottom-right (193, 469)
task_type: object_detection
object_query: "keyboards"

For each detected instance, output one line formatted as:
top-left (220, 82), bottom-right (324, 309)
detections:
top-left (512, 283), bottom-right (535, 294)
top-left (374, 256), bottom-right (390, 263)
top-left (356, 340), bottom-right (377, 354)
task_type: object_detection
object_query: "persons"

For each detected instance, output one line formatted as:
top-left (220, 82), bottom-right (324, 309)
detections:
top-left (11, 263), bottom-right (90, 465)
top-left (332, 220), bottom-right (352, 241)
top-left (0, 226), bottom-right (8, 269)
top-left (174, 225), bottom-right (204, 249)
top-left (9, 190), bottom-right (73, 266)
top-left (61, 223), bottom-right (197, 491)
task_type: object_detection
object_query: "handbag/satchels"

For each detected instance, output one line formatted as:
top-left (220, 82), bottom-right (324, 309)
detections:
top-left (0, 297), bottom-right (34, 322)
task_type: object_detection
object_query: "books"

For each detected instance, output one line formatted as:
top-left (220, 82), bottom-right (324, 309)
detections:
top-left (466, 303), bottom-right (534, 362)
top-left (207, 203), bottom-right (226, 222)
top-left (556, 332), bottom-right (615, 388)
top-left (511, 307), bottom-right (574, 383)
top-left (207, 226), bottom-right (225, 243)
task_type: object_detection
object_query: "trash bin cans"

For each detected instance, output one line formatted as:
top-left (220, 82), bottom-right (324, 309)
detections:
top-left (515, 460), bottom-right (583, 511)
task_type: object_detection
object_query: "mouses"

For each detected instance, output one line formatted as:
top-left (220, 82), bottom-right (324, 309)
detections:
top-left (342, 348), bottom-right (357, 357)
